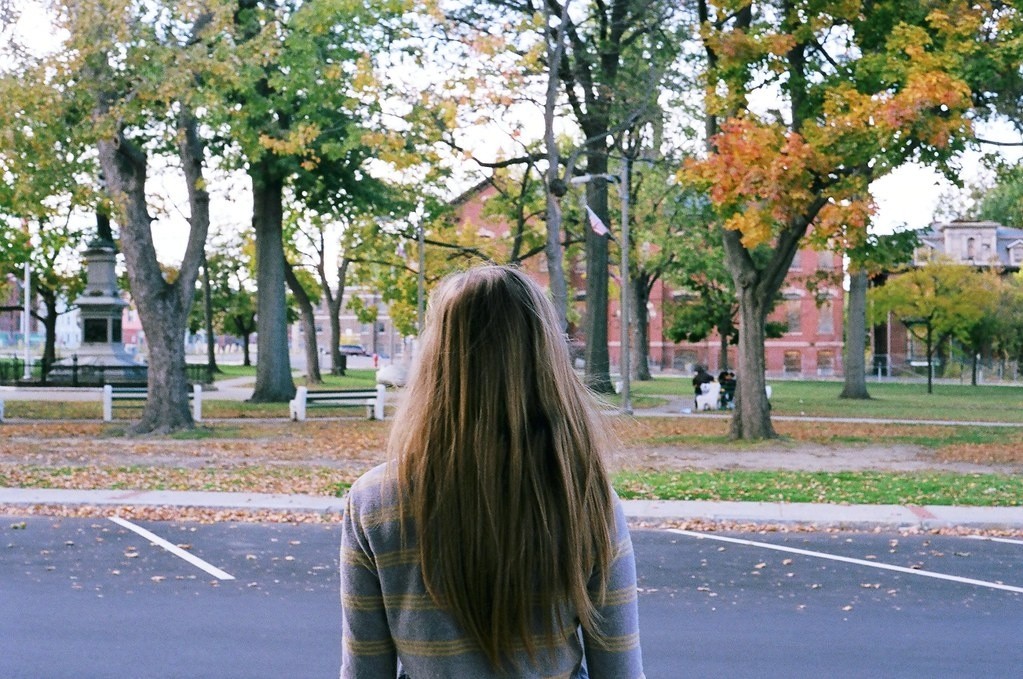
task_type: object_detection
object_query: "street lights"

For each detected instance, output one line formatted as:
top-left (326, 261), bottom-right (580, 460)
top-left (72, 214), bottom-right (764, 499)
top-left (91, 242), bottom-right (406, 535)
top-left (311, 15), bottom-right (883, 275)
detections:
top-left (571, 174), bottom-right (634, 416)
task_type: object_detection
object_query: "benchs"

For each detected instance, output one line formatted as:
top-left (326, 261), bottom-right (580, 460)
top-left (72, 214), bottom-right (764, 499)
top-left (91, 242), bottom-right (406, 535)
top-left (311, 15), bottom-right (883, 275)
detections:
top-left (102, 383), bottom-right (203, 421)
top-left (696, 382), bottom-right (722, 410)
top-left (289, 384), bottom-right (386, 421)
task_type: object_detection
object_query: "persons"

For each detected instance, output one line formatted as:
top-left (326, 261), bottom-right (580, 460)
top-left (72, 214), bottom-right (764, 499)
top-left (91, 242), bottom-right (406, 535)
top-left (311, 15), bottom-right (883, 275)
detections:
top-left (340, 265), bottom-right (647, 679)
top-left (718, 371), bottom-right (737, 410)
top-left (693, 369), bottom-right (714, 409)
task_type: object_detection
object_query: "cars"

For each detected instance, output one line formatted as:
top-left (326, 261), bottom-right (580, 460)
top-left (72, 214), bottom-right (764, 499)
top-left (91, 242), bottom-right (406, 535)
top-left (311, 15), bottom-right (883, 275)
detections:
top-left (325, 345), bottom-right (368, 356)
